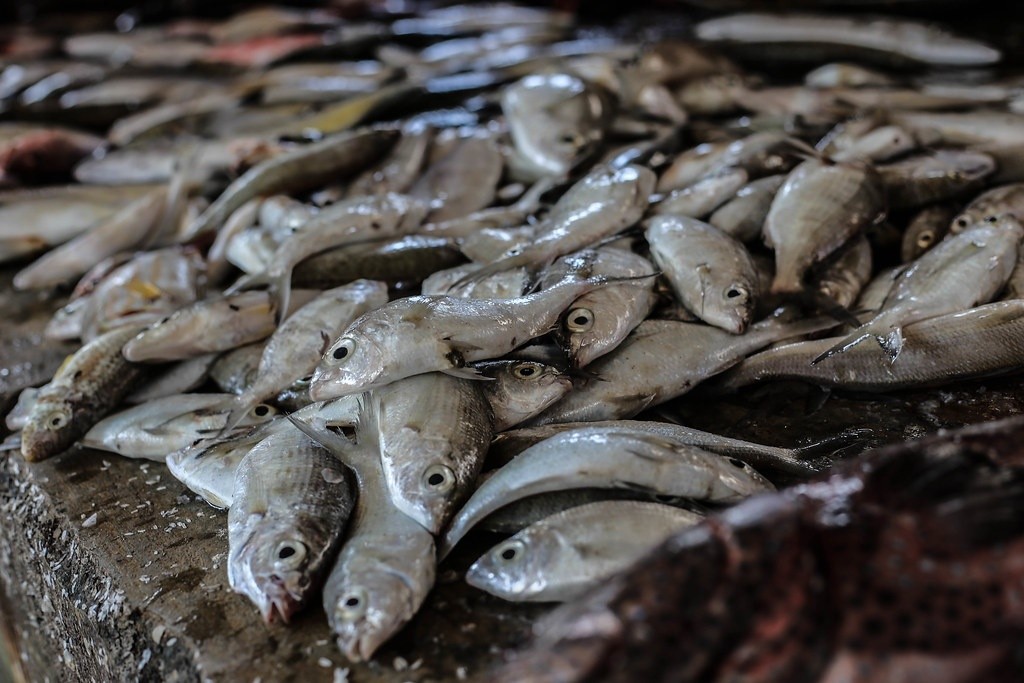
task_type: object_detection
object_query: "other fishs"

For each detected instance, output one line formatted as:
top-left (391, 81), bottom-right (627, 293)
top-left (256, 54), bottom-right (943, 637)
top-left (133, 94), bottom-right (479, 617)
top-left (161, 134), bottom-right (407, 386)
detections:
top-left (0, 1), bottom-right (1024, 668)
